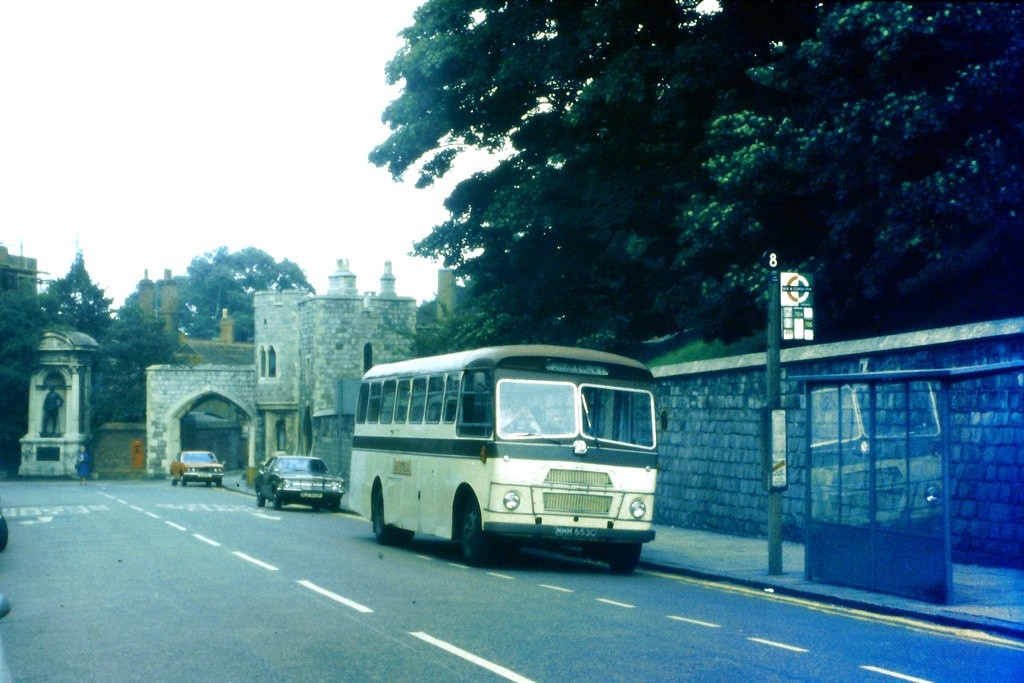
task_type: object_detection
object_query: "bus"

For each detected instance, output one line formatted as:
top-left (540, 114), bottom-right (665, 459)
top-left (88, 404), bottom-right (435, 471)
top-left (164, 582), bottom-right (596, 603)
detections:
top-left (809, 385), bottom-right (943, 527)
top-left (348, 345), bottom-right (669, 574)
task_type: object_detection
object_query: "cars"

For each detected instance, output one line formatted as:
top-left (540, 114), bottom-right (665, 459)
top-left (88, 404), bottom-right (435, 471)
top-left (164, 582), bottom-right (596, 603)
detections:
top-left (253, 455), bottom-right (345, 513)
top-left (170, 451), bottom-right (225, 487)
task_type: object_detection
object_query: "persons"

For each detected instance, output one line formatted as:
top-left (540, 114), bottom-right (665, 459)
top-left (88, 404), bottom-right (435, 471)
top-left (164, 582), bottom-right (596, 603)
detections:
top-left (75, 446), bottom-right (90, 486)
top-left (42, 386), bottom-right (64, 434)
top-left (500, 393), bottom-right (541, 435)
